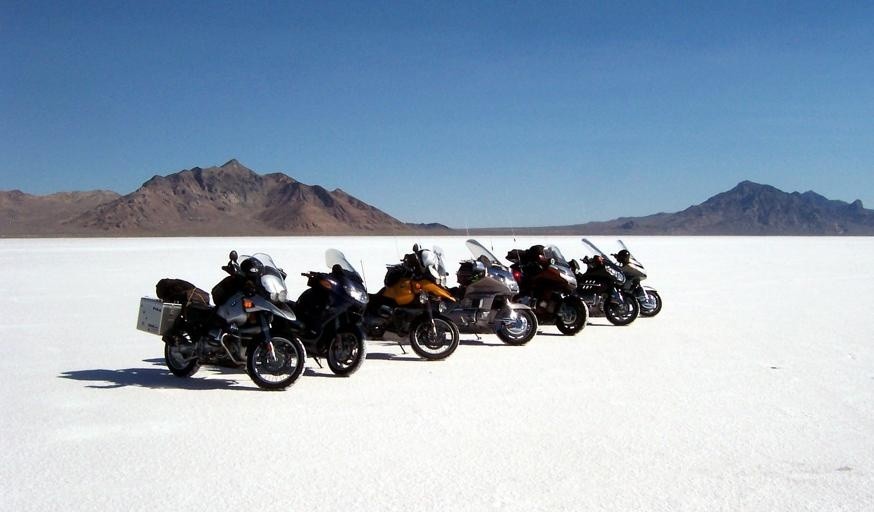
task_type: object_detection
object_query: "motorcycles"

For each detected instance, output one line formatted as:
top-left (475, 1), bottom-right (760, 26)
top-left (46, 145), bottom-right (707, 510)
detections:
top-left (567, 237), bottom-right (646, 325)
top-left (134, 250), bottom-right (307, 393)
top-left (217, 248), bottom-right (370, 376)
top-left (502, 243), bottom-right (593, 335)
top-left (362, 242), bottom-right (462, 360)
top-left (446, 240), bottom-right (540, 346)
top-left (606, 240), bottom-right (660, 318)
